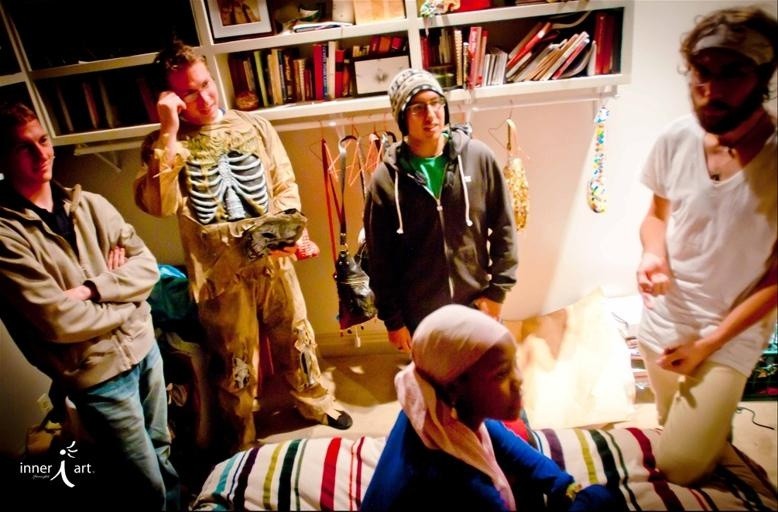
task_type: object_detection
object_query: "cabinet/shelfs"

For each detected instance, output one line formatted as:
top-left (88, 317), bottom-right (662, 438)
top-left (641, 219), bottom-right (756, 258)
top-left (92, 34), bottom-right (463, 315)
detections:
top-left (0, 0), bottom-right (637, 148)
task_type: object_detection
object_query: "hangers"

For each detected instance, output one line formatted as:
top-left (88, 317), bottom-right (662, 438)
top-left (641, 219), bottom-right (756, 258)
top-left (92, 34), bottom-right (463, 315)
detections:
top-left (308, 100), bottom-right (533, 189)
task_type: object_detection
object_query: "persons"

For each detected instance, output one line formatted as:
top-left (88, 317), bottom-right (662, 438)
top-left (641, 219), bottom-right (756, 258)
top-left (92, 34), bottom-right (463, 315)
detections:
top-left (636, 4), bottom-right (778, 488)
top-left (358, 302), bottom-right (622, 512)
top-left (361, 65), bottom-right (534, 431)
top-left (130, 35), bottom-right (355, 452)
top-left (0, 98), bottom-right (183, 512)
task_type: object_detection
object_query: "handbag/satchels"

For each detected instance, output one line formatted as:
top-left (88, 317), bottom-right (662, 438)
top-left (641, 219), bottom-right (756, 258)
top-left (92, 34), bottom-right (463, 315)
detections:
top-left (335, 242), bottom-right (378, 330)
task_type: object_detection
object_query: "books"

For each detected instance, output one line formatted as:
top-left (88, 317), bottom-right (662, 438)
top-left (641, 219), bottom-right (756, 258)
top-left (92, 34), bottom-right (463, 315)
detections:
top-left (39, 60), bottom-right (168, 136)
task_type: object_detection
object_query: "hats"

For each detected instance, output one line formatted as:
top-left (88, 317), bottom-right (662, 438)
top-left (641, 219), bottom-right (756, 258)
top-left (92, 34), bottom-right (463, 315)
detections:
top-left (389, 68), bottom-right (449, 136)
top-left (690, 13), bottom-right (774, 64)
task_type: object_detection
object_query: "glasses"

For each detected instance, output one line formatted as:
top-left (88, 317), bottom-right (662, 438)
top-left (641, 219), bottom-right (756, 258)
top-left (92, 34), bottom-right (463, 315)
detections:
top-left (406, 97), bottom-right (446, 115)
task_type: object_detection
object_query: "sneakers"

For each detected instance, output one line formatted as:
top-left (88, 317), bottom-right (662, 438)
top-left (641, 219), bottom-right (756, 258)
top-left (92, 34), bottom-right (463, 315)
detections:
top-left (328, 411), bottom-right (352, 430)
